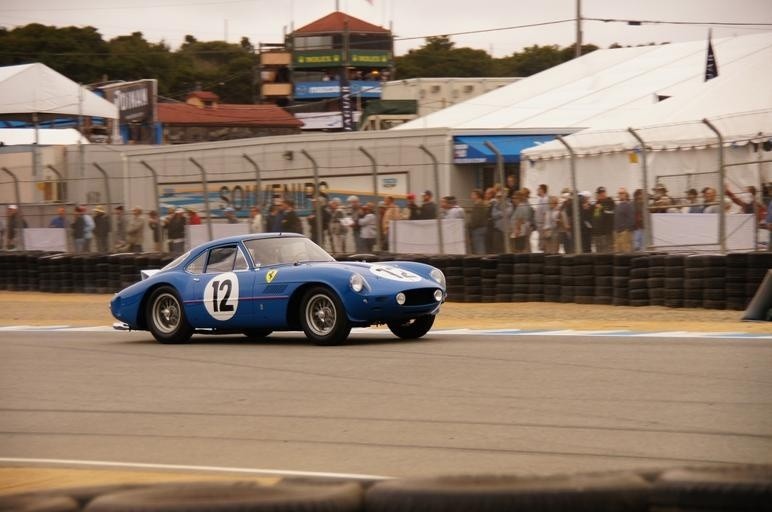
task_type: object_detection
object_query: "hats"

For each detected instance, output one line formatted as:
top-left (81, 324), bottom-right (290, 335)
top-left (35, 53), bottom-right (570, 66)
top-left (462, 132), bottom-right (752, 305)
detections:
top-left (560, 184), bottom-right (757, 206)
top-left (271, 190), bottom-right (457, 212)
top-left (8, 202), bottom-right (236, 214)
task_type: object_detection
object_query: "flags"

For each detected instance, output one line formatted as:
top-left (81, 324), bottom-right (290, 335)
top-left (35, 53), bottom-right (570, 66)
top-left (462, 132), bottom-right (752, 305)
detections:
top-left (706, 39), bottom-right (717, 80)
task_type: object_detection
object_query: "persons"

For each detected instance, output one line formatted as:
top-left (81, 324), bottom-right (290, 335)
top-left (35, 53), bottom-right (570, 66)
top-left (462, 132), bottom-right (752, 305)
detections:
top-left (441, 173), bottom-right (645, 254)
top-left (47, 203), bottom-right (202, 254)
top-left (222, 206), bottom-right (241, 224)
top-left (5, 204), bottom-right (29, 251)
top-left (306, 191), bottom-right (439, 255)
top-left (649, 182), bottom-right (771, 229)
top-left (247, 198), bottom-right (302, 234)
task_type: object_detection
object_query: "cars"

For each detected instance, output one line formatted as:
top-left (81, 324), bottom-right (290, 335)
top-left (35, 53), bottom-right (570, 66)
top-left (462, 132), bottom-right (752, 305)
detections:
top-left (109, 233), bottom-right (448, 343)
